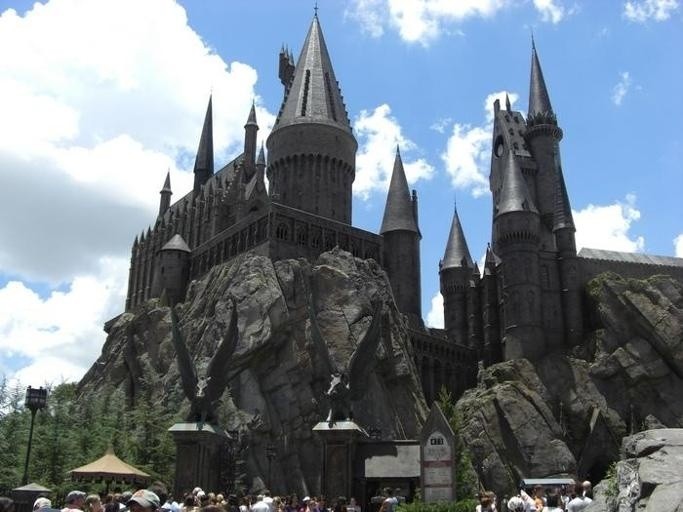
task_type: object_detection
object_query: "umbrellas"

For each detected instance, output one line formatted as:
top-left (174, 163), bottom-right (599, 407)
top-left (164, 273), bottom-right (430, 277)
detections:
top-left (66, 442), bottom-right (151, 494)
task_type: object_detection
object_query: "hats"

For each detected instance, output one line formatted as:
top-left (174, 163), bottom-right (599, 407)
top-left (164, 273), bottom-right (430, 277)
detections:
top-left (125, 488), bottom-right (161, 511)
top-left (507, 496), bottom-right (525, 510)
top-left (67, 490), bottom-right (86, 501)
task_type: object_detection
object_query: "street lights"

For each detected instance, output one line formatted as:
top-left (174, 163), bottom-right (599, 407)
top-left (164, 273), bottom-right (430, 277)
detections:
top-left (22, 385), bottom-right (47, 487)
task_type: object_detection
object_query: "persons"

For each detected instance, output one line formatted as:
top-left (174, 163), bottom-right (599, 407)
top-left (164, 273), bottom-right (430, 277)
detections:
top-left (371, 487), bottom-right (406, 512)
top-left (474, 479), bottom-right (594, 512)
top-left (0, 481), bottom-right (362, 512)
